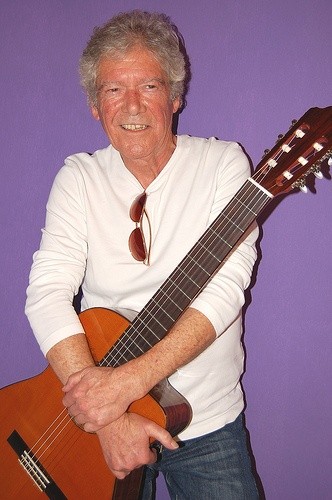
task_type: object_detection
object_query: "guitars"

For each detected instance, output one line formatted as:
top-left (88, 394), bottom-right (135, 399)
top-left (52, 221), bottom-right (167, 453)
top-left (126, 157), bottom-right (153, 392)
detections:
top-left (0, 100), bottom-right (332, 500)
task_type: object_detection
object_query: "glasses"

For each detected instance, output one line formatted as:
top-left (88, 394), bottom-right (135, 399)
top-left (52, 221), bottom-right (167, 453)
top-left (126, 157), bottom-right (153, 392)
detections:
top-left (128, 189), bottom-right (152, 266)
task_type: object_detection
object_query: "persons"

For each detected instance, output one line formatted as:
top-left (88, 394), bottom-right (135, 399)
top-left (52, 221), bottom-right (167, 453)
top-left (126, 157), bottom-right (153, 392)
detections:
top-left (24, 11), bottom-right (267, 500)
